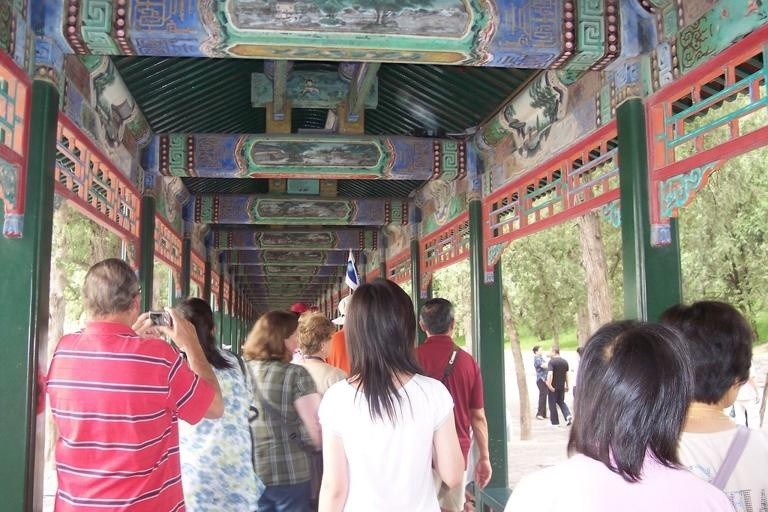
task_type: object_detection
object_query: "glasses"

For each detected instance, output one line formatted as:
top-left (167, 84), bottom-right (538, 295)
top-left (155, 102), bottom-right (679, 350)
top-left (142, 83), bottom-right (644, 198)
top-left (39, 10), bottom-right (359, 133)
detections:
top-left (138, 284), bottom-right (143, 296)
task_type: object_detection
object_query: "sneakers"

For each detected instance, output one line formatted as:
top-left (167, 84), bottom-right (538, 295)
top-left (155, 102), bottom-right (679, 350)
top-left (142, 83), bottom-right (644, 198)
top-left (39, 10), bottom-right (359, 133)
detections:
top-left (564, 415), bottom-right (574, 429)
top-left (535, 413), bottom-right (548, 421)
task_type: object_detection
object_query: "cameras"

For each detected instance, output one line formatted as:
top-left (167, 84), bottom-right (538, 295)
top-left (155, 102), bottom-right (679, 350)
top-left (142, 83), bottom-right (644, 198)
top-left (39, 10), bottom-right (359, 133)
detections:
top-left (149, 310), bottom-right (172, 328)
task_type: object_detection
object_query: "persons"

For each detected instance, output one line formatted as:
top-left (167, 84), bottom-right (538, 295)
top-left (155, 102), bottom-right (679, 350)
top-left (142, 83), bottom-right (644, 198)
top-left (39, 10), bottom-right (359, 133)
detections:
top-left (499, 319), bottom-right (738, 512)
top-left (533, 345), bottom-right (549, 419)
top-left (545, 343), bottom-right (573, 428)
top-left (659, 300), bottom-right (768, 511)
